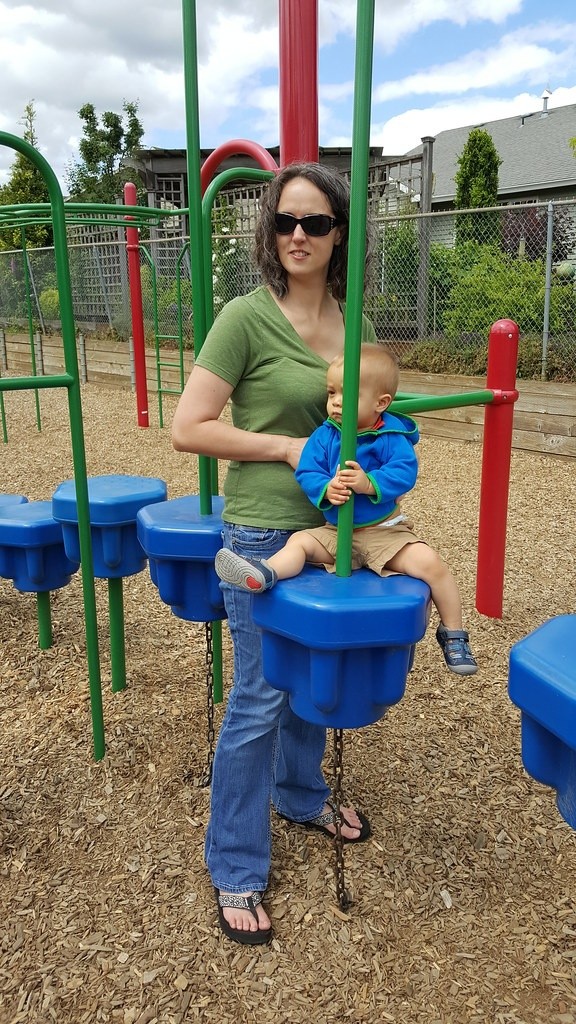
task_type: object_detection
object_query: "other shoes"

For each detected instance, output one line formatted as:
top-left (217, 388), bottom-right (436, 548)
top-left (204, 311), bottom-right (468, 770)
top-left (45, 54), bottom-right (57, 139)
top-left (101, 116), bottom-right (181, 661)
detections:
top-left (436, 623), bottom-right (478, 673)
top-left (215, 549), bottom-right (277, 594)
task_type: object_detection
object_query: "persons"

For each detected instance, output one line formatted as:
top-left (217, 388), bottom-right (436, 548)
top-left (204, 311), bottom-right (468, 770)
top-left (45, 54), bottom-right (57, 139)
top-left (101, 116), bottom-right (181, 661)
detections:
top-left (171, 161), bottom-right (376, 944)
top-left (216, 341), bottom-right (479, 676)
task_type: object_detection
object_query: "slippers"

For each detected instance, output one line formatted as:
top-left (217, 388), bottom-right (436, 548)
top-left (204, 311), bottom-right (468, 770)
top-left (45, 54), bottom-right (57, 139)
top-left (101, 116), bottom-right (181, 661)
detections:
top-left (276, 801), bottom-right (370, 841)
top-left (215, 885), bottom-right (272, 943)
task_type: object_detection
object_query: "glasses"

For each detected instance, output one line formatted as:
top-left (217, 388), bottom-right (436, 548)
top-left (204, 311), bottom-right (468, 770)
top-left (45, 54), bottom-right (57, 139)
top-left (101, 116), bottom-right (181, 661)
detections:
top-left (273, 212), bottom-right (339, 237)
